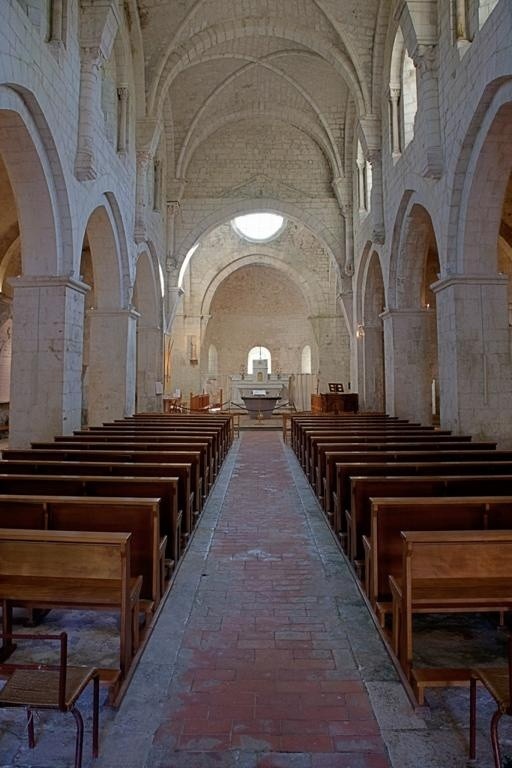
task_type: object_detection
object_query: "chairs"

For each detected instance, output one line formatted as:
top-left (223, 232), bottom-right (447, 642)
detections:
top-left (465, 663), bottom-right (510, 768)
top-left (1, 631), bottom-right (101, 768)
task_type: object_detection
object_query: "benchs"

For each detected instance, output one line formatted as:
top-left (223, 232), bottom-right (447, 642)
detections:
top-left (281, 415), bottom-right (512, 710)
top-left (1, 414), bottom-right (243, 706)
